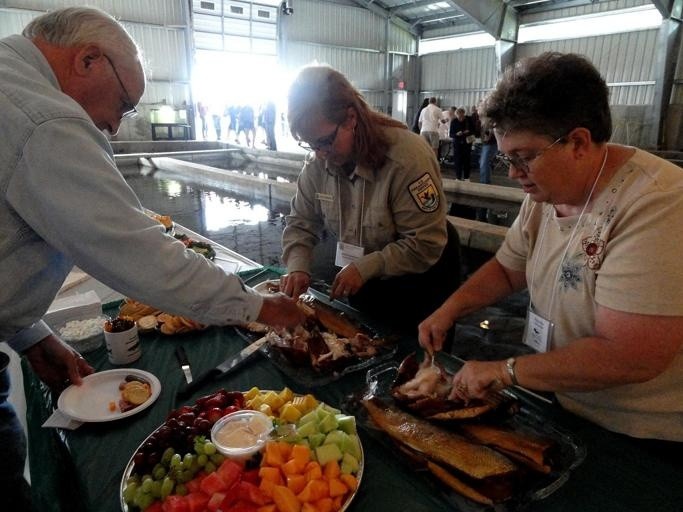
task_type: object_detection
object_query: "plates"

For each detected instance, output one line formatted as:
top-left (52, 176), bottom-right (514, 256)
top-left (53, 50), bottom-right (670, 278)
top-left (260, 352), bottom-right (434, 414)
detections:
top-left (57, 368), bottom-right (162, 422)
top-left (119, 389), bottom-right (365, 512)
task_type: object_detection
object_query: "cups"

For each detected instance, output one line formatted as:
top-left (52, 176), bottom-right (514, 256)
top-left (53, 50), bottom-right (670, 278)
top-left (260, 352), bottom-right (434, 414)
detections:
top-left (103, 319), bottom-right (143, 366)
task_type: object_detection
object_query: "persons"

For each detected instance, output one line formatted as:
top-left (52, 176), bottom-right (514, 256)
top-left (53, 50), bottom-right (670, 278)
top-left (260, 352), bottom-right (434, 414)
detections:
top-left (413, 97), bottom-right (500, 185)
top-left (418, 49), bottom-right (683, 505)
top-left (194, 97), bottom-right (277, 152)
top-left (282, 67), bottom-right (461, 357)
top-left (1, 8), bottom-right (310, 415)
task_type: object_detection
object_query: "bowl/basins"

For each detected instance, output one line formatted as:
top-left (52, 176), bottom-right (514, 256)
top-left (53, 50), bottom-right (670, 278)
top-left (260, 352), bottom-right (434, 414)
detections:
top-left (211, 409), bottom-right (274, 464)
top-left (53, 313), bottom-right (112, 354)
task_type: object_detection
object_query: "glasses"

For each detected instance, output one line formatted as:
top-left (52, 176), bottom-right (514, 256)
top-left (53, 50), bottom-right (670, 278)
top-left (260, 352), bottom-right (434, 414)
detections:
top-left (495, 137), bottom-right (563, 169)
top-left (295, 124), bottom-right (338, 151)
top-left (103, 54), bottom-right (138, 119)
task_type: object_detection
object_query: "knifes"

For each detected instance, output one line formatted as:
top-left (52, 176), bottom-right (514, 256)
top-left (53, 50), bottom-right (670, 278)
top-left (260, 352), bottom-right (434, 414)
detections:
top-left (175, 342), bottom-right (193, 384)
top-left (176, 336), bottom-right (266, 403)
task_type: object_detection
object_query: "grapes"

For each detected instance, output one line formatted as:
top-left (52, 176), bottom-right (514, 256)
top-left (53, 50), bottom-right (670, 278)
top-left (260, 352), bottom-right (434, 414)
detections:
top-left (125, 408), bottom-right (224, 509)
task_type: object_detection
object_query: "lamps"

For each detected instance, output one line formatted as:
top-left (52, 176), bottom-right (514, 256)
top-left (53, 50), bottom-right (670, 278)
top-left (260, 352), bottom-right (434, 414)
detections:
top-left (282, 0), bottom-right (294, 16)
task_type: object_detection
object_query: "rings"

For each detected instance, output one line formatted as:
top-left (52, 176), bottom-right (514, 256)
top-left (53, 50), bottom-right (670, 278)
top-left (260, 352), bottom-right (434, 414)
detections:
top-left (456, 382), bottom-right (467, 394)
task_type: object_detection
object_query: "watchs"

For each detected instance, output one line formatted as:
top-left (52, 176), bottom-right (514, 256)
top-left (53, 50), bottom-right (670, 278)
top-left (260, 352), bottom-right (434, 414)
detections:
top-left (507, 356), bottom-right (520, 387)
